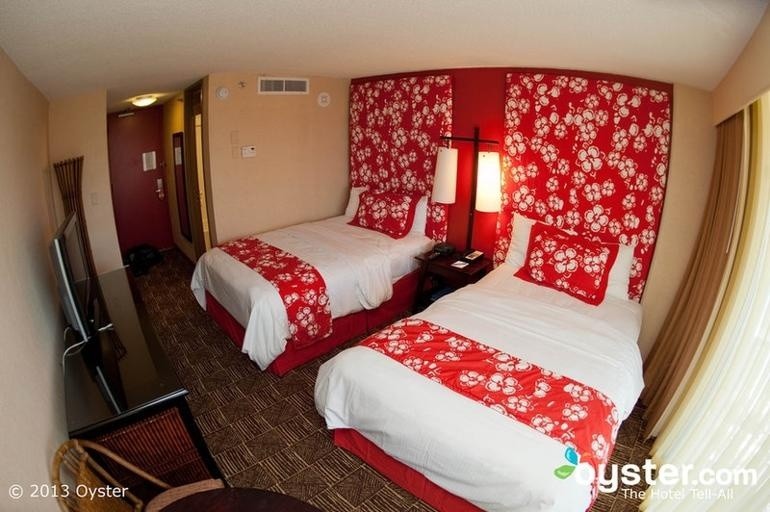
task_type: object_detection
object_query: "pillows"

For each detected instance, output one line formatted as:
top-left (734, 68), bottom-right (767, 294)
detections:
top-left (344, 188), bottom-right (429, 237)
top-left (347, 191), bottom-right (425, 239)
top-left (505, 213), bottom-right (635, 300)
top-left (512, 222), bottom-right (620, 307)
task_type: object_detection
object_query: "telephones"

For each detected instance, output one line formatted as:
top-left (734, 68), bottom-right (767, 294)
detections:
top-left (460, 249), bottom-right (484, 264)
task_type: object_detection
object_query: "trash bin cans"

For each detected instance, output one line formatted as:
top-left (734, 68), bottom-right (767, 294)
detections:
top-left (430, 287), bottom-right (453, 301)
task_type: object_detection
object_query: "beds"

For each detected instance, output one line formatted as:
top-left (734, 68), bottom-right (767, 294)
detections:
top-left (188, 76), bottom-right (453, 378)
top-left (314, 73), bottom-right (672, 512)
top-left (158, 487), bottom-right (322, 512)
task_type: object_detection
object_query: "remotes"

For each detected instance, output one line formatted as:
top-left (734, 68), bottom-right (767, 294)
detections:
top-left (428, 252), bottom-right (440, 260)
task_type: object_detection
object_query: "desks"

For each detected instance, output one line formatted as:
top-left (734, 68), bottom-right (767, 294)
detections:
top-left (64, 264), bottom-right (220, 480)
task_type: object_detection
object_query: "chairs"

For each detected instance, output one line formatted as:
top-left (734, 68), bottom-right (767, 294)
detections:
top-left (51, 440), bottom-right (225, 512)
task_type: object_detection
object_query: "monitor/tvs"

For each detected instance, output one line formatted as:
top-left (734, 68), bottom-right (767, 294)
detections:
top-left (82, 291), bottom-right (128, 416)
top-left (49, 212), bottom-right (97, 343)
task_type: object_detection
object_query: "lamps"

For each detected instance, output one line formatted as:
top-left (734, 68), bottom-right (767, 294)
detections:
top-left (132, 95), bottom-right (156, 107)
top-left (474, 144), bottom-right (502, 214)
top-left (432, 136), bottom-right (458, 205)
